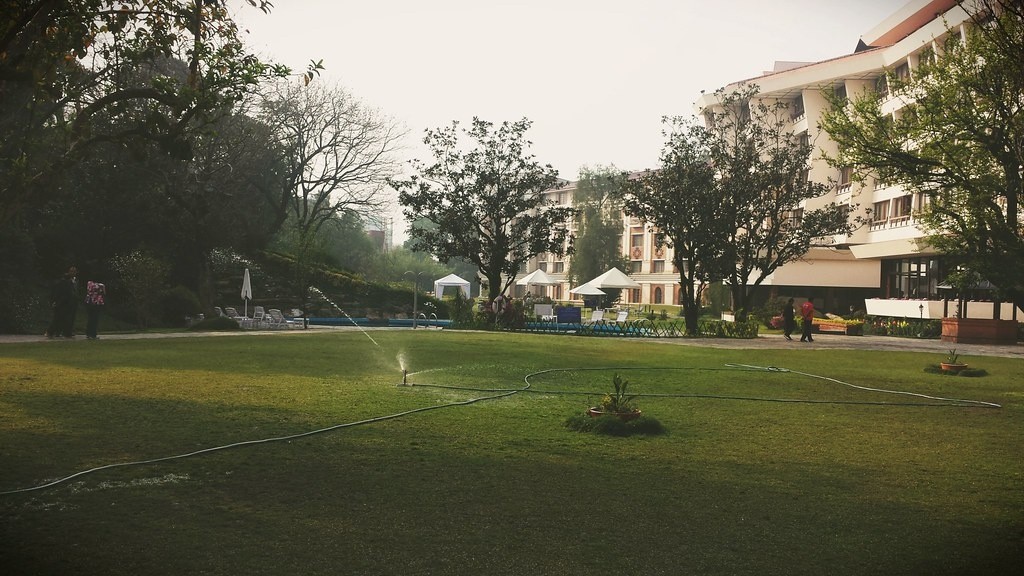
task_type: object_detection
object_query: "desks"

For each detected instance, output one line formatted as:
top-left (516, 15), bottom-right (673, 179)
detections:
top-left (605, 308), bottom-right (618, 314)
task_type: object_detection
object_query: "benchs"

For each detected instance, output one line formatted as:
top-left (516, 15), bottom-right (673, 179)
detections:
top-left (226, 308), bottom-right (241, 321)
top-left (251, 306), bottom-right (267, 329)
top-left (215, 307), bottom-right (226, 318)
top-left (267, 309), bottom-right (294, 328)
top-left (293, 318), bottom-right (310, 328)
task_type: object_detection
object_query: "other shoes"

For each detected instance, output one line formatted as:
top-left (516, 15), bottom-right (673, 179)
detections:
top-left (800, 339), bottom-right (808, 342)
top-left (784, 334), bottom-right (790, 340)
top-left (809, 339), bottom-right (814, 342)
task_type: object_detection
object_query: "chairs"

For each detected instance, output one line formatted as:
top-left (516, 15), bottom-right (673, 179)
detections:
top-left (634, 306), bottom-right (645, 316)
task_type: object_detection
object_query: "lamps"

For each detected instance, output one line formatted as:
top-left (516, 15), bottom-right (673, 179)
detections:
top-left (918, 303), bottom-right (924, 315)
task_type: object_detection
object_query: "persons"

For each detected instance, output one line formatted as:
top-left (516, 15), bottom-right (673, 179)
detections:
top-left (800, 296), bottom-right (815, 342)
top-left (782, 297), bottom-right (795, 340)
top-left (44, 264), bottom-right (108, 340)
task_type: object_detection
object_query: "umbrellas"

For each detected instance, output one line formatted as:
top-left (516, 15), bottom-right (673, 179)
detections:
top-left (240, 269), bottom-right (253, 319)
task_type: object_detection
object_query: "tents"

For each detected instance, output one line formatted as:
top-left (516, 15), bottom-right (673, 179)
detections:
top-left (434, 274), bottom-right (471, 307)
top-left (568, 283), bottom-right (607, 315)
top-left (516, 269), bottom-right (562, 308)
top-left (586, 267), bottom-right (642, 319)
top-left (935, 267), bottom-right (1002, 319)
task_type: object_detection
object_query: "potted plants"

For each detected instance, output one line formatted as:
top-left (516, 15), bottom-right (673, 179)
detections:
top-left (940, 344), bottom-right (968, 372)
top-left (588, 373), bottom-right (641, 423)
